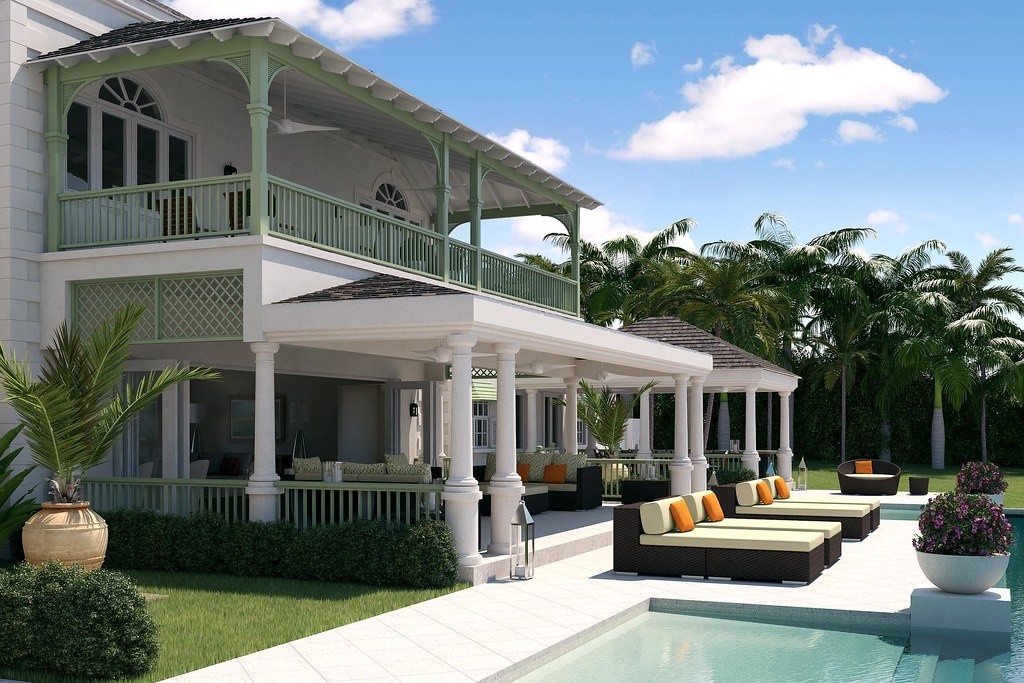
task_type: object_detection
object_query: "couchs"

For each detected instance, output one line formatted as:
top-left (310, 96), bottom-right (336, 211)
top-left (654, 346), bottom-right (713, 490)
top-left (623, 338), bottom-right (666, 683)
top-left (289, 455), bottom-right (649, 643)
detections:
top-left (282, 465), bottom-right (602, 551)
top-left (199, 454), bottom-right (292, 523)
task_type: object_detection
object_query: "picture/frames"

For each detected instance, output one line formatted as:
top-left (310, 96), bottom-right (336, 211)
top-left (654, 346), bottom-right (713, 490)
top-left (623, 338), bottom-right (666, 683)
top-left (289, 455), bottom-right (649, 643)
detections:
top-left (227, 394), bottom-right (285, 444)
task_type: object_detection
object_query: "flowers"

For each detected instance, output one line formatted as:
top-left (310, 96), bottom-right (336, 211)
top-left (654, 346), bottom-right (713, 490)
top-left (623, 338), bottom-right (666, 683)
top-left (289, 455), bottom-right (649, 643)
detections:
top-left (911, 488), bottom-right (1014, 559)
top-left (955, 461), bottom-right (1008, 496)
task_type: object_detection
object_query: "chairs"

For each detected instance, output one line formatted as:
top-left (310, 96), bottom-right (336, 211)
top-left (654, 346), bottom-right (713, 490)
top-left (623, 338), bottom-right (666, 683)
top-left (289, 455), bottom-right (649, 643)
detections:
top-left (155, 196), bottom-right (229, 243)
top-left (222, 188), bottom-right (298, 238)
top-left (138, 460), bottom-right (211, 513)
top-left (709, 450), bottom-right (729, 471)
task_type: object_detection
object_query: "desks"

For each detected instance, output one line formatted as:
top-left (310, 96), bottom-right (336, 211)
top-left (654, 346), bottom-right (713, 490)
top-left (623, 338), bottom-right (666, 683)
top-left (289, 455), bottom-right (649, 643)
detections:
top-left (909, 476), bottom-right (929, 495)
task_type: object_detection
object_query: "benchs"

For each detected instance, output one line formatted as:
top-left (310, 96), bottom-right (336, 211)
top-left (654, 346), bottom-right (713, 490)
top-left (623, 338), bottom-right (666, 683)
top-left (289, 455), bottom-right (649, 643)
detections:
top-left (837, 459), bottom-right (901, 496)
top-left (613, 475), bottom-right (880, 584)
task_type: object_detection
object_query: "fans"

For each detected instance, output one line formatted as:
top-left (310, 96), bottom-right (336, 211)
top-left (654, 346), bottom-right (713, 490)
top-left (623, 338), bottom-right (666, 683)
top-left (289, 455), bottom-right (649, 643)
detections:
top-left (269, 75), bottom-right (341, 137)
top-left (402, 183), bottom-right (466, 214)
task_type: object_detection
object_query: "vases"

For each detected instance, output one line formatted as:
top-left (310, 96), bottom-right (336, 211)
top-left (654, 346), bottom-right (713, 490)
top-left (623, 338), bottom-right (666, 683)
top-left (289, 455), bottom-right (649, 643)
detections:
top-left (987, 492), bottom-right (1006, 506)
top-left (916, 551), bottom-right (1012, 594)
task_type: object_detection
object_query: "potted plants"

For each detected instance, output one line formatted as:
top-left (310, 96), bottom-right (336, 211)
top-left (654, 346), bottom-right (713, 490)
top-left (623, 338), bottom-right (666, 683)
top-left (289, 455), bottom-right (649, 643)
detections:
top-left (0, 298), bottom-right (225, 573)
top-left (549, 377), bottom-right (663, 480)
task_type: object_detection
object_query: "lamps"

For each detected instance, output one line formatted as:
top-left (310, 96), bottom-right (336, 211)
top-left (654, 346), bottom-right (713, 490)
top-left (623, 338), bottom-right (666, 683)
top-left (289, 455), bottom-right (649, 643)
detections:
top-left (410, 402), bottom-right (418, 417)
top-left (189, 403), bottom-right (206, 454)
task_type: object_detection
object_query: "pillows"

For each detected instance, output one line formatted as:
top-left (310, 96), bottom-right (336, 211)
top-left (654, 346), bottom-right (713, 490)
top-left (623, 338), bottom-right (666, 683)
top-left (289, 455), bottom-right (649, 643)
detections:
top-left (756, 480), bottom-right (773, 505)
top-left (854, 461), bottom-right (872, 474)
top-left (552, 454), bottom-right (587, 483)
top-left (484, 453), bottom-right (519, 482)
top-left (544, 464), bottom-right (567, 483)
top-left (201, 453), bottom-right (294, 481)
top-left (702, 493), bottom-right (724, 522)
top-left (383, 453), bottom-right (409, 464)
top-left (670, 498), bottom-right (695, 532)
top-left (341, 461), bottom-right (387, 475)
top-left (292, 457), bottom-right (323, 474)
top-left (386, 463), bottom-right (433, 483)
top-left (518, 453), bottom-right (553, 483)
top-left (774, 477), bottom-right (790, 499)
